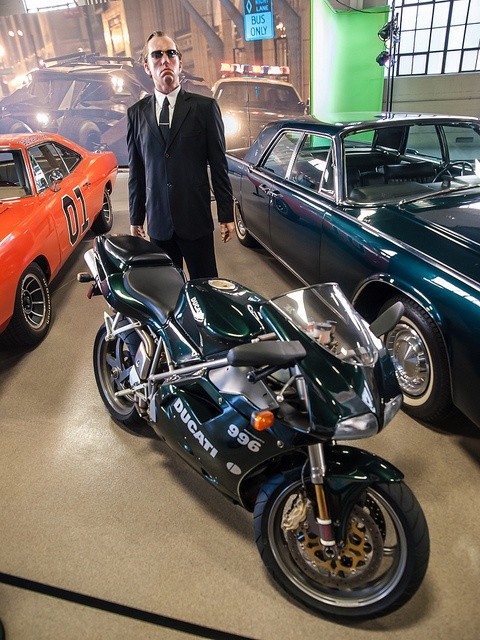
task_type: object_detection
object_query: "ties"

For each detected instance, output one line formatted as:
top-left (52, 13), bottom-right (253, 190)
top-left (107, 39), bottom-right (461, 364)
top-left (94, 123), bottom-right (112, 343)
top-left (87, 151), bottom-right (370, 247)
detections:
top-left (159, 97), bottom-right (169, 144)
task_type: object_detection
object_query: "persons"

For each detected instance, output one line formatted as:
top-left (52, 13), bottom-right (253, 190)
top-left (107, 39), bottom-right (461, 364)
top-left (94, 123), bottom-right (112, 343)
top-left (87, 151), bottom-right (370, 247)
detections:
top-left (127, 30), bottom-right (236, 283)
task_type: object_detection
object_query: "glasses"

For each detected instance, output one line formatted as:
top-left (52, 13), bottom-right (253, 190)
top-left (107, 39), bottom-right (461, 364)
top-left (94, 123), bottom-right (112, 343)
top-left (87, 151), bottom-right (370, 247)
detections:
top-left (148, 50), bottom-right (178, 58)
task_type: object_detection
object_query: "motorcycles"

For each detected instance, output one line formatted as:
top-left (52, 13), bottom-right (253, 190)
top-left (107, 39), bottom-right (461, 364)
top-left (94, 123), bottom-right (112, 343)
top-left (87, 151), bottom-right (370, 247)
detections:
top-left (76, 232), bottom-right (431, 625)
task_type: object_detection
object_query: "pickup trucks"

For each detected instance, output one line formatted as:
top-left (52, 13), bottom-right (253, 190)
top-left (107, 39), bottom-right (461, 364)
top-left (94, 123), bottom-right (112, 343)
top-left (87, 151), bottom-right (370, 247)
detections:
top-left (215, 111), bottom-right (480, 431)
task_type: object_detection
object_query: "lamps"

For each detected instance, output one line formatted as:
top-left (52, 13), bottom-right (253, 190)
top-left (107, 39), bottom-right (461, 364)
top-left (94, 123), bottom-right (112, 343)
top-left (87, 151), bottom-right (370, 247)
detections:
top-left (378, 21), bottom-right (397, 43)
top-left (375, 51), bottom-right (389, 67)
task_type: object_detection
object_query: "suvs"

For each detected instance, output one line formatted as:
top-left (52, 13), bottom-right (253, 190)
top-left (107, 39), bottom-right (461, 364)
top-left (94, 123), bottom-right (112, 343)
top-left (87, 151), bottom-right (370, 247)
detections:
top-left (210, 62), bottom-right (310, 151)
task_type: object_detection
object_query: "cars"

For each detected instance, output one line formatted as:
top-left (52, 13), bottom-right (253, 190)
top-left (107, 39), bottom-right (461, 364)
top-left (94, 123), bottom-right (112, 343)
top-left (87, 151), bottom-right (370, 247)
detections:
top-left (0, 131), bottom-right (118, 346)
